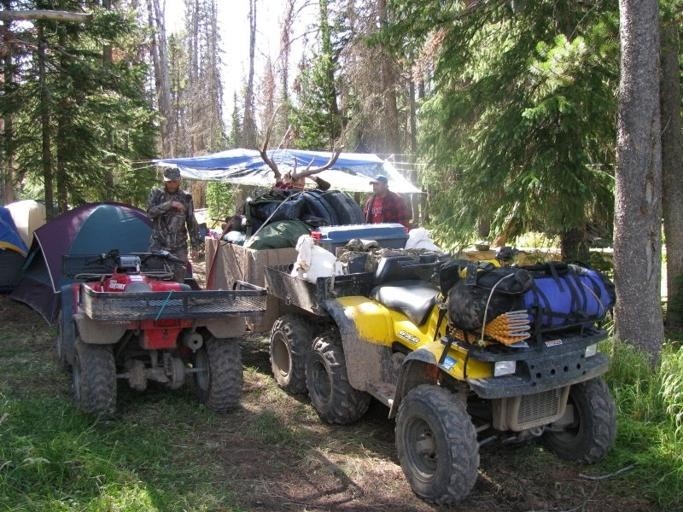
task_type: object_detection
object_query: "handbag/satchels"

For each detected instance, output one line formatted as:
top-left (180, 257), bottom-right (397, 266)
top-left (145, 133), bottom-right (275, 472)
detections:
top-left (447, 261), bottom-right (618, 333)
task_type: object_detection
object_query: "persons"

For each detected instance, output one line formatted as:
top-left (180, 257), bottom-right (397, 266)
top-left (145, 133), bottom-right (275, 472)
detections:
top-left (146, 169), bottom-right (200, 266)
top-left (362, 175), bottom-right (410, 234)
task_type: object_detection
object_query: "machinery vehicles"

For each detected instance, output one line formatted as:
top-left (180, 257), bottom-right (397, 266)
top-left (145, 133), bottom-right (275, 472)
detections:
top-left (268, 223), bottom-right (618, 507)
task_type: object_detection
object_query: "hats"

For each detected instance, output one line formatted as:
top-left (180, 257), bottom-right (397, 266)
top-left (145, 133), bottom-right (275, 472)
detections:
top-left (369, 174), bottom-right (389, 185)
top-left (163, 167), bottom-right (181, 182)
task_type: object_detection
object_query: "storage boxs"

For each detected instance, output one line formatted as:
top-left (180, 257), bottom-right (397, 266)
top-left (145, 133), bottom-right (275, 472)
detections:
top-left (316, 223), bottom-right (409, 257)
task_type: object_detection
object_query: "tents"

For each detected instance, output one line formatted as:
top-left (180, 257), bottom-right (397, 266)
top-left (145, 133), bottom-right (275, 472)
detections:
top-left (0, 198), bottom-right (154, 329)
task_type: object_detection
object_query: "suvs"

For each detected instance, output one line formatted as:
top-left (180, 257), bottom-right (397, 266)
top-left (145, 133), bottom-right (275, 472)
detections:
top-left (55, 247), bottom-right (271, 419)
